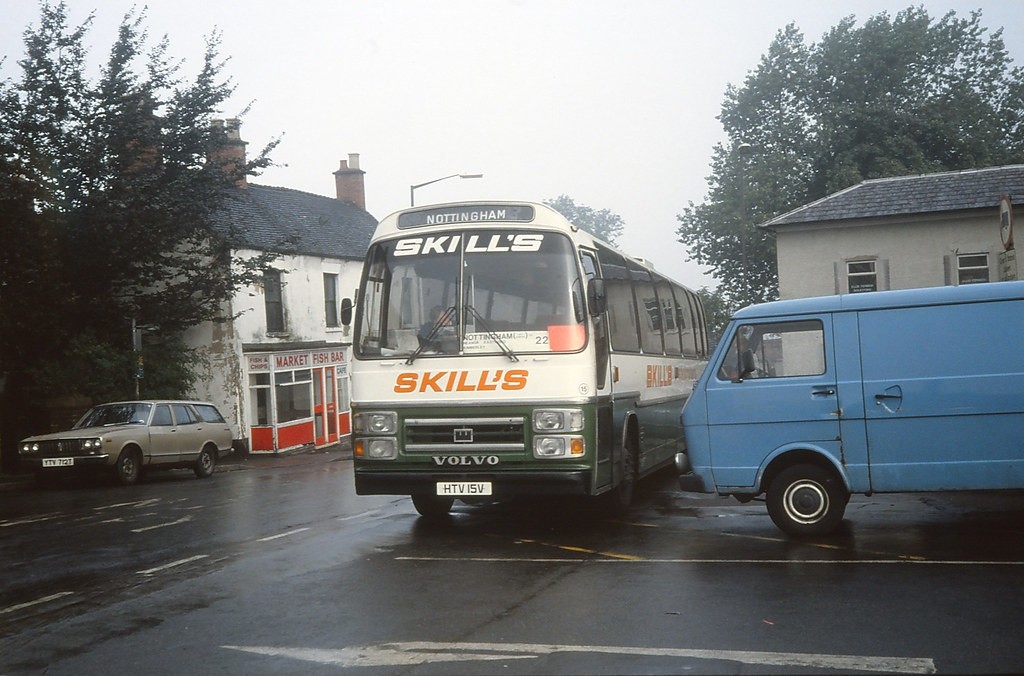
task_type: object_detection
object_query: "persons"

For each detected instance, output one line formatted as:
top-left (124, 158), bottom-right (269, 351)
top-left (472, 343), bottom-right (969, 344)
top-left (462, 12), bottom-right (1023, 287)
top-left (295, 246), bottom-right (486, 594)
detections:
top-left (430, 304), bottom-right (457, 327)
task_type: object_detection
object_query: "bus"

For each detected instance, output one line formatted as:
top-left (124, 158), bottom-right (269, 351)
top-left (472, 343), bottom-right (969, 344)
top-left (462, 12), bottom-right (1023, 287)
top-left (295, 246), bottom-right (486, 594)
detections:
top-left (336, 201), bottom-right (712, 529)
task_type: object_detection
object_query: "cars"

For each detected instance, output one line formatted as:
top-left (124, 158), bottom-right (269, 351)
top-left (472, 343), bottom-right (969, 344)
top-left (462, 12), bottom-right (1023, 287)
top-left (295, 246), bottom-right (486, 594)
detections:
top-left (18, 399), bottom-right (236, 485)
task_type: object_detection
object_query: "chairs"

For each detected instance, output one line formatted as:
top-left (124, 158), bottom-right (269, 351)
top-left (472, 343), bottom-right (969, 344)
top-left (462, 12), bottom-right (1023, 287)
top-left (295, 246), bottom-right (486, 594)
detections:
top-left (448, 317), bottom-right (567, 331)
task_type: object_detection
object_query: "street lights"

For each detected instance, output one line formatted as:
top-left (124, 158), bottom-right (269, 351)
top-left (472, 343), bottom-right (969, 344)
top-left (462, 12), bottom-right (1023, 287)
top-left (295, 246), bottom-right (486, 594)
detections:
top-left (409, 172), bottom-right (482, 208)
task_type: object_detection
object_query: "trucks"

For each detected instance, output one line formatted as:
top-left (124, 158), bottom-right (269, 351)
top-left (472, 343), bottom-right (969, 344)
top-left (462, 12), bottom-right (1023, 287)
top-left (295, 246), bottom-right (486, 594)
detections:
top-left (678, 278), bottom-right (1022, 537)
